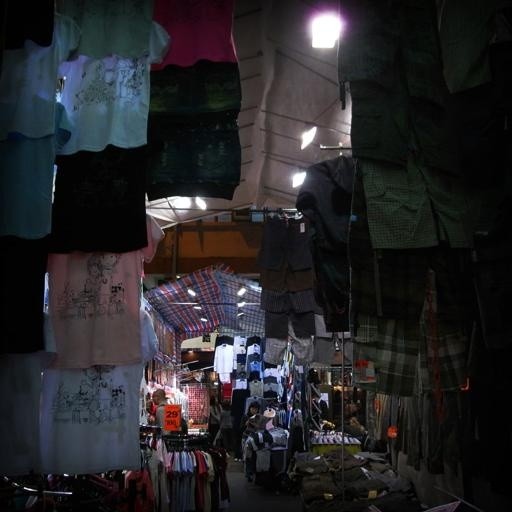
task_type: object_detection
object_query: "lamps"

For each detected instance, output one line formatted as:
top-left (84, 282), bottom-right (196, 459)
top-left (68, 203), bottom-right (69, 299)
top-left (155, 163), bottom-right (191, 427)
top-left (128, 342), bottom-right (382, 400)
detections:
top-left (292, 170), bottom-right (308, 189)
top-left (300, 120), bottom-right (317, 149)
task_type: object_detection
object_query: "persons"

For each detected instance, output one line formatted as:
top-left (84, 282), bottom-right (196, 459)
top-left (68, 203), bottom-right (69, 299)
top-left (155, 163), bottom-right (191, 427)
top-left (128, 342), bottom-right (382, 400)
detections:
top-left (151, 388), bottom-right (170, 426)
top-left (233, 400), bottom-right (261, 462)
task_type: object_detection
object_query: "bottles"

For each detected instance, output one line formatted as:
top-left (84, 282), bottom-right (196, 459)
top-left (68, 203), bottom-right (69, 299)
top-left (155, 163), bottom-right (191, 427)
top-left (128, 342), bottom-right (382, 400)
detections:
top-left (159, 408), bottom-right (164, 428)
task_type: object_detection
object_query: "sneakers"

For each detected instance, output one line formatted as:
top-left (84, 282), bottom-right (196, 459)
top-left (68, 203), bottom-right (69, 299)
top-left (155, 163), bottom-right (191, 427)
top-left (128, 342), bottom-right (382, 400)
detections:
top-left (311, 431), bottom-right (361, 444)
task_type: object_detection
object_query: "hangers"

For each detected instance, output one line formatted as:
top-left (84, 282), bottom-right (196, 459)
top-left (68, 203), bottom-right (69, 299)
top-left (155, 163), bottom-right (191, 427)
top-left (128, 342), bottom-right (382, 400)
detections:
top-left (160, 434), bottom-right (215, 452)
